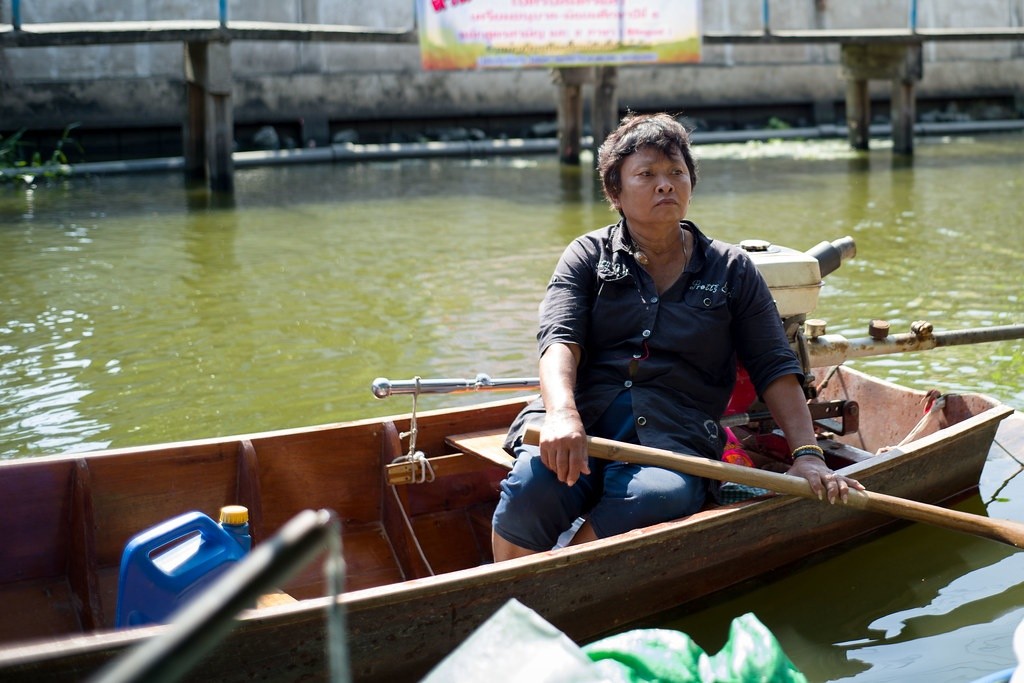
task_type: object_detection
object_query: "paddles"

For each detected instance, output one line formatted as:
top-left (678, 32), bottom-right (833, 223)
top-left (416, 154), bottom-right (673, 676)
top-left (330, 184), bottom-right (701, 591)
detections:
top-left (519, 420), bottom-right (1023, 550)
top-left (384, 450), bottom-right (496, 486)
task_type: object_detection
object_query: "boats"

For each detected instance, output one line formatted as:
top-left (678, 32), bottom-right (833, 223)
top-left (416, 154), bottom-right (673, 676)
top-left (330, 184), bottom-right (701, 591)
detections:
top-left (0, 230), bottom-right (1016, 683)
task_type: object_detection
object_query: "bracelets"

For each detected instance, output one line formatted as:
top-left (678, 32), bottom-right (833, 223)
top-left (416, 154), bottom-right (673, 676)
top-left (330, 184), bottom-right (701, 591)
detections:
top-left (791, 444), bottom-right (825, 461)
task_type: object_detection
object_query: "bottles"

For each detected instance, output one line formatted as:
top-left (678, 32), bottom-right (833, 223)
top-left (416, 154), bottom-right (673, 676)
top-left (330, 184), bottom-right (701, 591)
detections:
top-left (117, 504), bottom-right (251, 630)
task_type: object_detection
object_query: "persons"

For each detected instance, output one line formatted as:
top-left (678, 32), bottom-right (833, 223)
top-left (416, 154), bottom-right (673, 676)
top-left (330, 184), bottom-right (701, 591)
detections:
top-left (492, 112), bottom-right (865, 562)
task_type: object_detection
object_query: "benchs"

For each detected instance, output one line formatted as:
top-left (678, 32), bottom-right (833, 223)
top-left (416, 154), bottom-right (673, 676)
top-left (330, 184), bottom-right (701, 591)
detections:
top-left (442, 426), bottom-right (516, 490)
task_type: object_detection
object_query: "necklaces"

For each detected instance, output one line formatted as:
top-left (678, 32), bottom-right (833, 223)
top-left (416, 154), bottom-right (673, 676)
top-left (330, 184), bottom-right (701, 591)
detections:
top-left (632, 228), bottom-right (688, 274)
